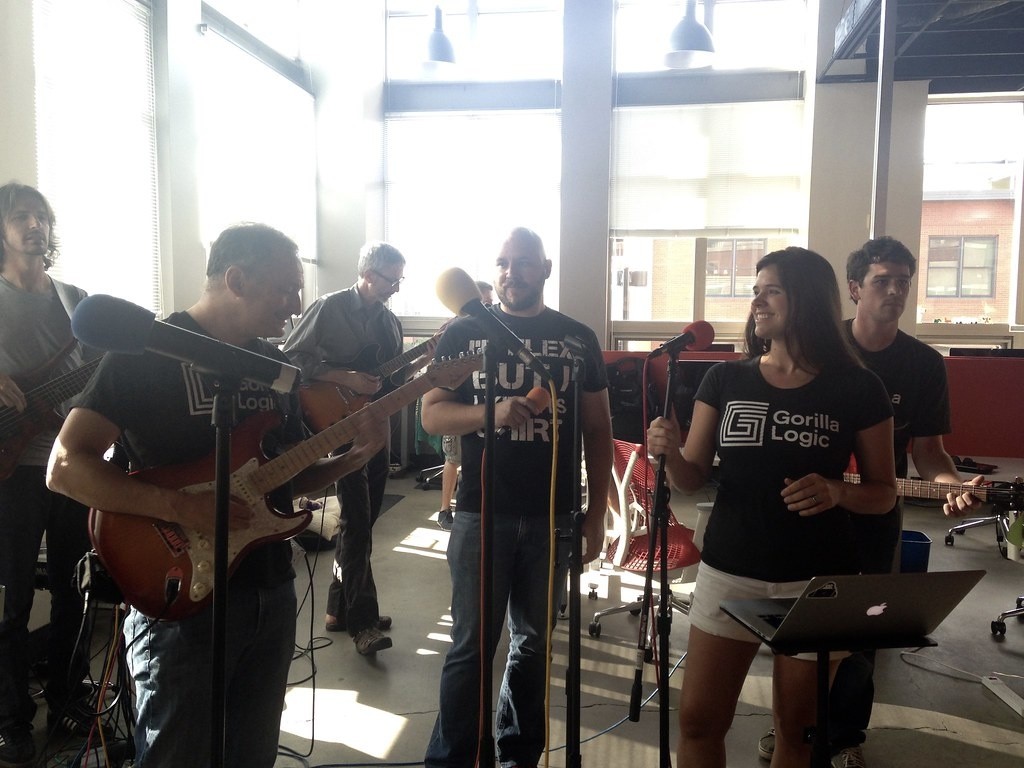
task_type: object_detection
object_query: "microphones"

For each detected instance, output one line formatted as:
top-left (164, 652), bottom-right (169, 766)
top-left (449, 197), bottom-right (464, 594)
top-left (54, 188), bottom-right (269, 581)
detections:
top-left (435, 267), bottom-right (550, 382)
top-left (72, 295), bottom-right (301, 396)
top-left (648, 321), bottom-right (715, 360)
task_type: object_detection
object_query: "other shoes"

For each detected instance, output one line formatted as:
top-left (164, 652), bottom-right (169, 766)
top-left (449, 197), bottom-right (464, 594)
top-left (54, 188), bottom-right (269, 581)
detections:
top-left (437, 508), bottom-right (454, 533)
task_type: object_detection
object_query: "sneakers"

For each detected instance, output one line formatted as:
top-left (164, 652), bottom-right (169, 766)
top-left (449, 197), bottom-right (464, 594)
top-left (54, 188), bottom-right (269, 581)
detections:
top-left (758, 724), bottom-right (774, 759)
top-left (46, 706), bottom-right (113, 739)
top-left (830, 743), bottom-right (865, 768)
top-left (0, 726), bottom-right (35, 762)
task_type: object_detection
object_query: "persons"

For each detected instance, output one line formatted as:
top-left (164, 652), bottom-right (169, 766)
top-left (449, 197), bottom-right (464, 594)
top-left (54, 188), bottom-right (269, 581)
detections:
top-left (44, 224), bottom-right (391, 768)
top-left (436, 282), bottom-right (493, 532)
top-left (282, 244), bottom-right (438, 657)
top-left (759, 238), bottom-right (985, 767)
top-left (646, 248), bottom-right (897, 768)
top-left (0, 182), bottom-right (112, 768)
top-left (420, 227), bottom-right (613, 768)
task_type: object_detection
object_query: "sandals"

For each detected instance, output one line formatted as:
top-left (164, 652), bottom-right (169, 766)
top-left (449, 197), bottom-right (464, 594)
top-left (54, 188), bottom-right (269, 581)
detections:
top-left (354, 627), bottom-right (392, 654)
top-left (325, 610), bottom-right (392, 632)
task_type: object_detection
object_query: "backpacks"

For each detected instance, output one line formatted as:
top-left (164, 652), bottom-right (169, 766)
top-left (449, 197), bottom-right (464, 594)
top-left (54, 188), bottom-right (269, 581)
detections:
top-left (601, 358), bottom-right (658, 442)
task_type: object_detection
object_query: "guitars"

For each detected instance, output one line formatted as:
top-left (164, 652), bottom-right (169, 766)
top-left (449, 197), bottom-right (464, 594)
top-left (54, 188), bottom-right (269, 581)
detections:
top-left (298, 301), bottom-right (492, 436)
top-left (1, 352), bottom-right (103, 476)
top-left (88, 344), bottom-right (486, 622)
top-left (843, 469), bottom-right (1024, 512)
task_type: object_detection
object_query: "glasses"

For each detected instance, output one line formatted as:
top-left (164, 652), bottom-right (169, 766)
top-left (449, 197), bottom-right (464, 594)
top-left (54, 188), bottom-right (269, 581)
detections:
top-left (369, 269), bottom-right (405, 286)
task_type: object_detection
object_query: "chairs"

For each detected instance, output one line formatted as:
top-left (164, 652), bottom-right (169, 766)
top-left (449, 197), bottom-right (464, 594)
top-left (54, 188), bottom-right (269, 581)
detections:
top-left (588, 439), bottom-right (704, 663)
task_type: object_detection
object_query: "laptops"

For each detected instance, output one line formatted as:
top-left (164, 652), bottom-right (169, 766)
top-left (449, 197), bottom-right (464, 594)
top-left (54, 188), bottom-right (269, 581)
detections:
top-left (716, 568), bottom-right (987, 645)
